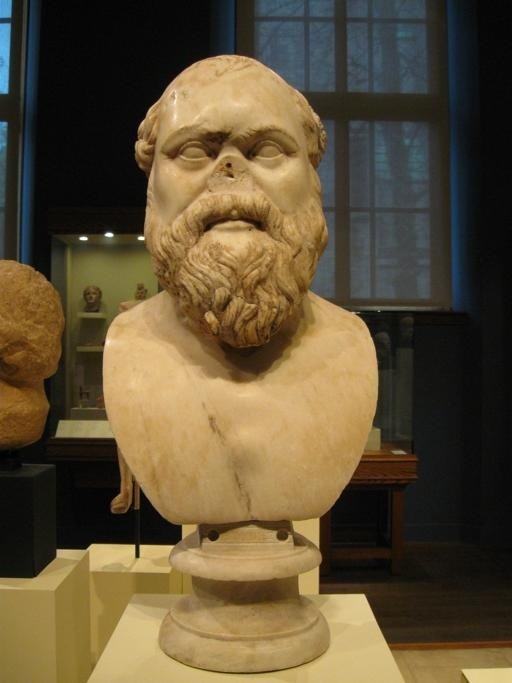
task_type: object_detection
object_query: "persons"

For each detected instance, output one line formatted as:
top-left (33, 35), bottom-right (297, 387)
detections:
top-left (135, 282), bottom-right (148, 301)
top-left (82, 285), bottom-right (102, 312)
top-left (99, 49), bottom-right (382, 542)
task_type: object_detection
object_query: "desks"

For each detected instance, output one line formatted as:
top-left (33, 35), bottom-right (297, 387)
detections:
top-left (320, 440), bottom-right (418, 576)
